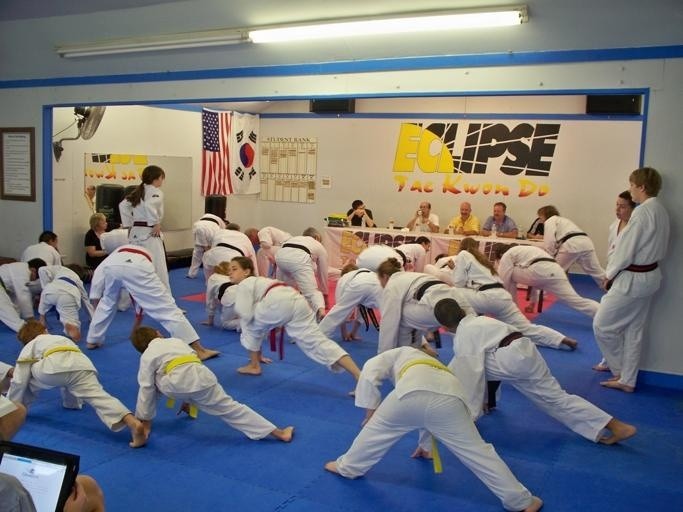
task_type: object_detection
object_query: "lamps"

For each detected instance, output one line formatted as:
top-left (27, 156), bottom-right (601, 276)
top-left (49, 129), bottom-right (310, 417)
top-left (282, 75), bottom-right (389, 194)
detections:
top-left (249, 3), bottom-right (542, 61)
top-left (59, 26), bottom-right (249, 59)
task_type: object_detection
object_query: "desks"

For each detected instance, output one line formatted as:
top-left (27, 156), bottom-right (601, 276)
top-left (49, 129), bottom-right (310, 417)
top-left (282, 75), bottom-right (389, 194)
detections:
top-left (321, 225), bottom-right (549, 315)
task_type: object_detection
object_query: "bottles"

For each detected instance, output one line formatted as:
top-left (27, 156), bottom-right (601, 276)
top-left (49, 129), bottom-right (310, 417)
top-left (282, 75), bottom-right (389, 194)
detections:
top-left (415, 219), bottom-right (420, 232)
top-left (449, 221), bottom-right (453, 236)
top-left (362, 215), bottom-right (365, 226)
top-left (492, 224), bottom-right (496, 238)
top-left (389, 217), bottom-right (393, 230)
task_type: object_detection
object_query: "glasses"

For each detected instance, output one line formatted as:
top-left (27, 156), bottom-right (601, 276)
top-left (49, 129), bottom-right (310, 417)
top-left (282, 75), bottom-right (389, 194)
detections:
top-left (421, 206), bottom-right (429, 209)
top-left (357, 205), bottom-right (365, 210)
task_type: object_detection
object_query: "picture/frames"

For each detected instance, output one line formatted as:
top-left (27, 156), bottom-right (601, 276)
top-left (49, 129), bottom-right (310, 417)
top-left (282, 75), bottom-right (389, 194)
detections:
top-left (0, 127), bottom-right (37, 202)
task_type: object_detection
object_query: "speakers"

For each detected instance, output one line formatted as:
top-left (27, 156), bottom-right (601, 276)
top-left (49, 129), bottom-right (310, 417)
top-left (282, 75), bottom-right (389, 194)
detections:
top-left (309, 99), bottom-right (355, 116)
top-left (586, 94), bottom-right (642, 116)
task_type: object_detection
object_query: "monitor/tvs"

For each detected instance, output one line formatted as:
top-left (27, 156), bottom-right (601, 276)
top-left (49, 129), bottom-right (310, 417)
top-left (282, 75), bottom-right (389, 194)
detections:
top-left (0, 440), bottom-right (81, 512)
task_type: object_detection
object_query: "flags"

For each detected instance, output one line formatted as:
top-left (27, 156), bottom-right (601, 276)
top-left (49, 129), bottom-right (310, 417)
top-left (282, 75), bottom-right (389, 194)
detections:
top-left (233, 111), bottom-right (261, 196)
top-left (198, 106), bottom-right (234, 197)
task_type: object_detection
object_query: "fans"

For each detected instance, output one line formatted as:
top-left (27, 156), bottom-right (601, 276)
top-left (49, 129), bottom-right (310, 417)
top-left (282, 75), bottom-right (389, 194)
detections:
top-left (54, 104), bottom-right (110, 163)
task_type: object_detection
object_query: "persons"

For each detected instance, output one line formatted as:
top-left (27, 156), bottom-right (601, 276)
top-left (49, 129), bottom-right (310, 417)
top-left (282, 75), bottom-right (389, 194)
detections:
top-left (593, 168), bottom-right (673, 393)
top-left (324, 345), bottom-right (544, 512)
top-left (319, 264), bottom-right (383, 340)
top-left (495, 243), bottom-right (601, 317)
top-left (526, 210), bottom-right (544, 241)
top-left (448, 237), bottom-right (578, 352)
top-left (5, 320), bottom-right (148, 448)
top-left (118, 164), bottom-right (186, 314)
top-left (1, 470), bottom-right (102, 512)
top-left (185, 212), bottom-right (228, 279)
top-left (406, 202), bottom-right (439, 233)
top-left (38, 264), bottom-right (95, 342)
top-left (227, 257), bottom-right (366, 398)
top-left (394, 237), bottom-right (431, 272)
top-left (200, 262), bottom-right (241, 332)
top-left (344, 199), bottom-right (377, 228)
top-left (271, 227), bottom-right (334, 321)
top-left (130, 326), bottom-right (295, 442)
top-left (0, 259), bottom-right (48, 332)
top-left (378, 257), bottom-right (478, 354)
top-left (0, 357), bottom-right (16, 393)
top-left (0, 397), bottom-right (28, 442)
top-left (202, 222), bottom-right (260, 286)
top-left (480, 202), bottom-right (521, 238)
top-left (244, 226), bottom-right (293, 277)
top-left (535, 205), bottom-right (609, 294)
top-left (424, 253), bottom-right (457, 285)
top-left (86, 244), bottom-right (220, 360)
top-left (86, 184), bottom-right (96, 211)
top-left (353, 243), bottom-right (408, 278)
top-left (431, 298), bottom-right (639, 446)
top-left (22, 230), bottom-right (63, 311)
top-left (85, 212), bottom-right (109, 267)
top-left (442, 202), bottom-right (479, 237)
top-left (592, 187), bottom-right (639, 371)
